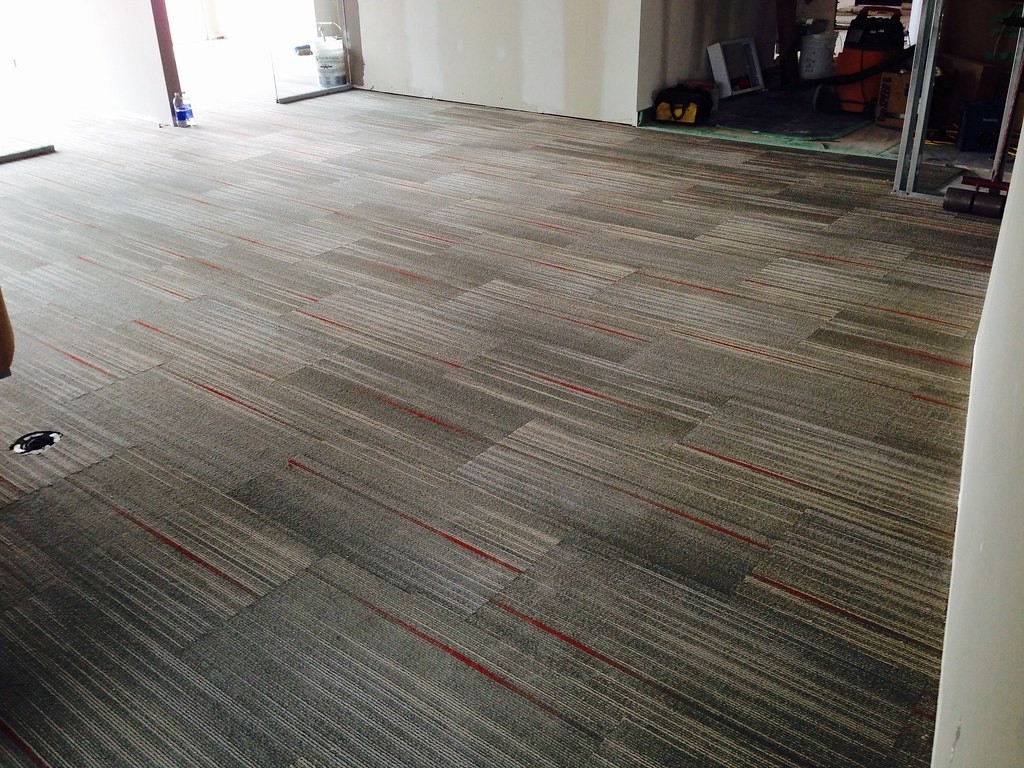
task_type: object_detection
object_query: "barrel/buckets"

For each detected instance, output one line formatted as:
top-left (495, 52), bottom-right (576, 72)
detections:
top-left (314, 36), bottom-right (351, 87)
top-left (799, 32), bottom-right (837, 78)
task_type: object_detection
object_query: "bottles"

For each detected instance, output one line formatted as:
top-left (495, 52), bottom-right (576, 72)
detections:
top-left (173, 93), bottom-right (186, 127)
top-left (182, 92), bottom-right (196, 126)
